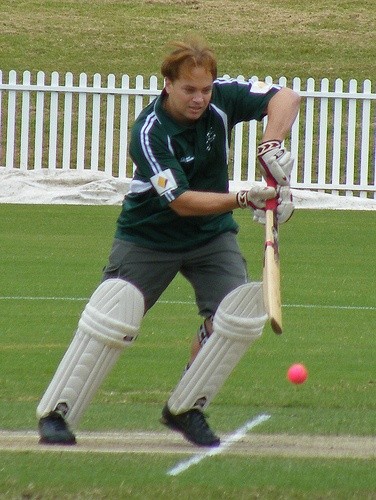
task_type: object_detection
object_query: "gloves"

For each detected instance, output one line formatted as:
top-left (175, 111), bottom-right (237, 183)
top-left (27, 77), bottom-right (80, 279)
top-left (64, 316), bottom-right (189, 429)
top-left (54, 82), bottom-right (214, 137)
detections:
top-left (237, 185), bottom-right (295, 224)
top-left (257, 140), bottom-right (294, 187)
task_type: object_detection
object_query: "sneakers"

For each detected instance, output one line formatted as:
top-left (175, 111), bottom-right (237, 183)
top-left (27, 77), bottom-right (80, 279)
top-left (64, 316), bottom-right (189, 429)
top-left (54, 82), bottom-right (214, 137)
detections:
top-left (38, 411), bottom-right (77, 445)
top-left (159, 402), bottom-right (221, 447)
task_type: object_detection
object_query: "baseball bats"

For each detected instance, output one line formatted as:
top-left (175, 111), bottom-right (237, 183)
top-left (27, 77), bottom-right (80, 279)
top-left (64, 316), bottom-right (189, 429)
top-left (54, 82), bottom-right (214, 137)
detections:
top-left (263, 177), bottom-right (284, 335)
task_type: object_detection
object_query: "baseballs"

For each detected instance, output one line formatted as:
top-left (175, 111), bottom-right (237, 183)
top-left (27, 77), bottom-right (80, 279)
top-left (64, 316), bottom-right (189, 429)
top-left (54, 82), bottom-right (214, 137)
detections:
top-left (287, 363), bottom-right (307, 384)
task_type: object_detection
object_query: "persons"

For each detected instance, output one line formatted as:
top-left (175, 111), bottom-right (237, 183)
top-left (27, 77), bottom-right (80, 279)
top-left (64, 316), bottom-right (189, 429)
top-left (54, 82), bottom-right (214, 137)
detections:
top-left (37, 33), bottom-right (301, 447)
